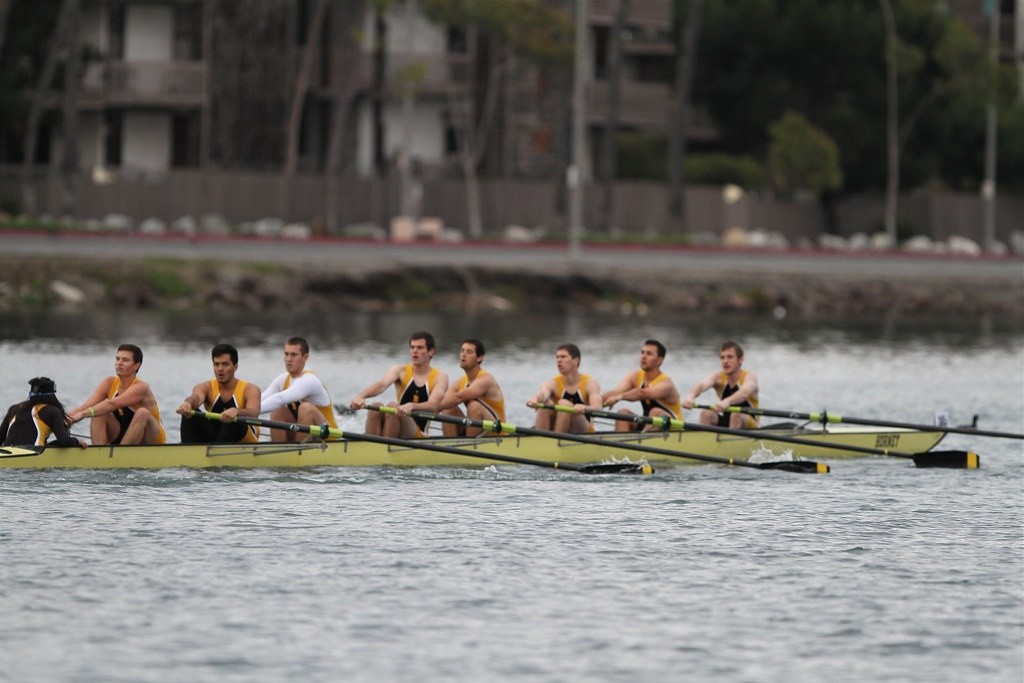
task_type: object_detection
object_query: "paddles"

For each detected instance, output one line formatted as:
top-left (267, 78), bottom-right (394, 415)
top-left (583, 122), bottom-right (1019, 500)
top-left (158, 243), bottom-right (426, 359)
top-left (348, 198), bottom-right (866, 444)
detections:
top-left (191, 410), bottom-right (656, 476)
top-left (536, 403), bottom-right (982, 469)
top-left (695, 403), bottom-right (1024, 441)
top-left (362, 405), bottom-right (831, 475)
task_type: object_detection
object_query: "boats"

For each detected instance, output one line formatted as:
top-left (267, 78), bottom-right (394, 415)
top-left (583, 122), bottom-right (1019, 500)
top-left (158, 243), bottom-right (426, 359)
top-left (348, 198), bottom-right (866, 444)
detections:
top-left (0, 413), bottom-right (952, 471)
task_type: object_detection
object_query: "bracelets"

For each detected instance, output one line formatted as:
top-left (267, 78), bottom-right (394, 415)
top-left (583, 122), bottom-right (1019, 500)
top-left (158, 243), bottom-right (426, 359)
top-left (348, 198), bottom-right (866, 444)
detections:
top-left (89, 407), bottom-right (94, 416)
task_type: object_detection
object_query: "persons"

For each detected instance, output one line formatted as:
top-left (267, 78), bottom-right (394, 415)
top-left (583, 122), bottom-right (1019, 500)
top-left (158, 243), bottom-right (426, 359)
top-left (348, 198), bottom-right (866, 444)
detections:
top-left (683, 342), bottom-right (760, 430)
top-left (601, 340), bottom-right (683, 432)
top-left (0, 376), bottom-right (88, 450)
top-left (529, 344), bottom-right (603, 434)
top-left (351, 331), bottom-right (449, 438)
top-left (260, 339), bottom-right (338, 441)
top-left (65, 344), bottom-right (167, 445)
top-left (439, 339), bottom-right (506, 436)
top-left (181, 344), bottom-right (260, 443)
